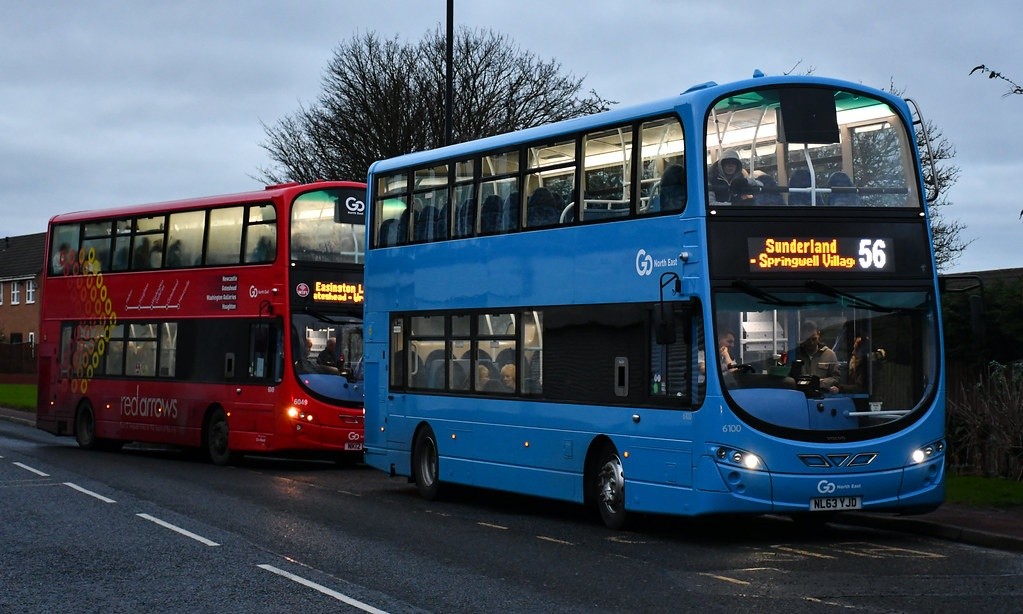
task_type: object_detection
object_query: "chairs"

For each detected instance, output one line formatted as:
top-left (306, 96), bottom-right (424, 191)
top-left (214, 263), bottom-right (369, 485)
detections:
top-left (379, 170), bottom-right (858, 244)
top-left (400, 348), bottom-right (541, 400)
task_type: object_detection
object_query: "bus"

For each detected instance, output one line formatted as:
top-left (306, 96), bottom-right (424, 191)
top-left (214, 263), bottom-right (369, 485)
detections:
top-left (35, 176), bottom-right (405, 466)
top-left (362, 70), bottom-right (990, 532)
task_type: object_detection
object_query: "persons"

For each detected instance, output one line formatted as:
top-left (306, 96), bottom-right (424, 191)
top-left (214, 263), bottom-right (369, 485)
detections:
top-left (250, 232), bottom-right (302, 262)
top-left (800, 321), bottom-right (880, 394)
top-left (52, 243), bottom-right (71, 274)
top-left (306, 337), bottom-right (345, 370)
top-left (136, 237), bottom-right (183, 268)
top-left (697, 327), bottom-right (738, 383)
top-left (465, 364), bottom-right (516, 392)
top-left (709, 148), bottom-right (755, 206)
